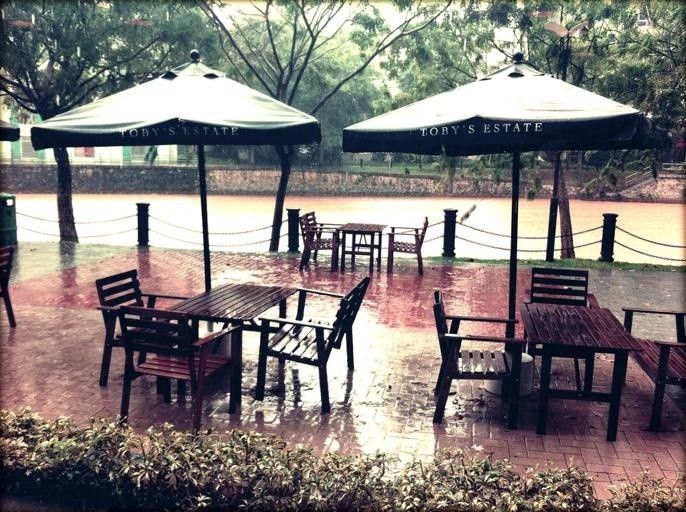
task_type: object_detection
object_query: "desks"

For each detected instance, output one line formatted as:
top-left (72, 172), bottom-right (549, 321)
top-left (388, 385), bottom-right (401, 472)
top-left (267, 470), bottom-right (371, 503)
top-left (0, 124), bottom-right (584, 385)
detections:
top-left (519, 302), bottom-right (645, 441)
top-left (337, 222), bottom-right (387, 273)
top-left (164, 282), bottom-right (297, 401)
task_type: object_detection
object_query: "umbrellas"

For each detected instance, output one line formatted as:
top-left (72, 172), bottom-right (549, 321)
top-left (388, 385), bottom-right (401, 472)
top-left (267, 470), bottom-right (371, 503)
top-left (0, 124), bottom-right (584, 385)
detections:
top-left (342, 51), bottom-right (655, 355)
top-left (29, 48), bottom-right (323, 334)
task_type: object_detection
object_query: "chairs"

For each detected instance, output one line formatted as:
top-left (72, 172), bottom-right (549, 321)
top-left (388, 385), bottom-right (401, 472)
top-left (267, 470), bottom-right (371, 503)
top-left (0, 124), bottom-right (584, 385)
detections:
top-left (433, 289), bottom-right (526, 429)
top-left (387, 216), bottom-right (428, 275)
top-left (256, 277), bottom-right (372, 415)
top-left (621, 307), bottom-right (686, 431)
top-left (119, 305), bottom-right (241, 431)
top-left (299, 211), bottom-right (344, 271)
top-left (531, 266), bottom-right (590, 390)
top-left (0, 247), bottom-right (16, 327)
top-left (96, 268), bottom-right (194, 387)
